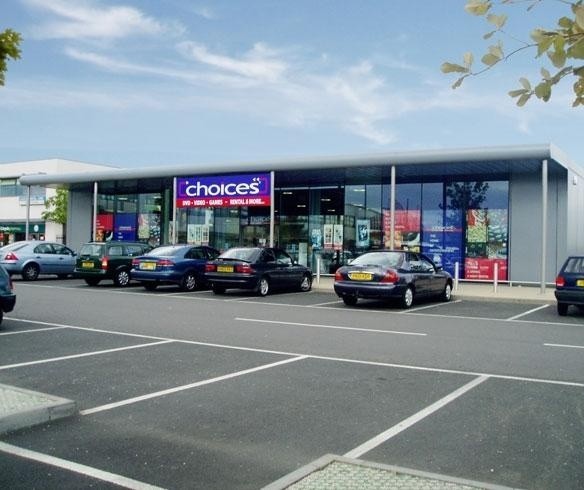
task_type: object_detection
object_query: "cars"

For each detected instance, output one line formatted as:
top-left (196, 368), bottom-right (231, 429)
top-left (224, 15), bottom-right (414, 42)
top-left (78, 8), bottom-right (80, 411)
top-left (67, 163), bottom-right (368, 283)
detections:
top-left (204, 247), bottom-right (312, 296)
top-left (553, 256), bottom-right (584, 315)
top-left (129, 243), bottom-right (222, 290)
top-left (335, 249), bottom-right (453, 307)
top-left (0, 240), bottom-right (76, 280)
top-left (74, 242), bottom-right (154, 286)
top-left (329, 250), bottom-right (353, 274)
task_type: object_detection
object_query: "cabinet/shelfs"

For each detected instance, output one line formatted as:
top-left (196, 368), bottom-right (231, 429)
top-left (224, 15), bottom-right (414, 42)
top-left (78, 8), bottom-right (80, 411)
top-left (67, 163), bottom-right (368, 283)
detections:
top-left (280, 222), bottom-right (330, 274)
